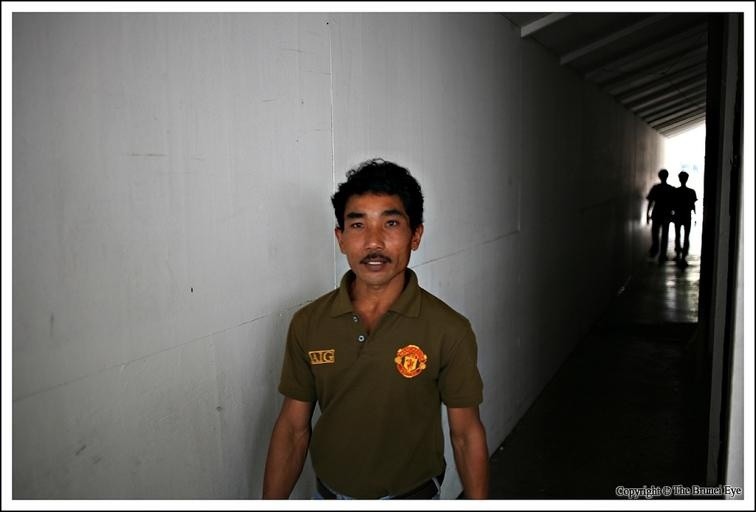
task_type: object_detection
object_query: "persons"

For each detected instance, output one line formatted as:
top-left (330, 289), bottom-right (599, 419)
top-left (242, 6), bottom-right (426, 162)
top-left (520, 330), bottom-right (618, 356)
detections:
top-left (262, 155), bottom-right (491, 500)
top-left (671, 171), bottom-right (697, 269)
top-left (645, 169), bottom-right (677, 261)
